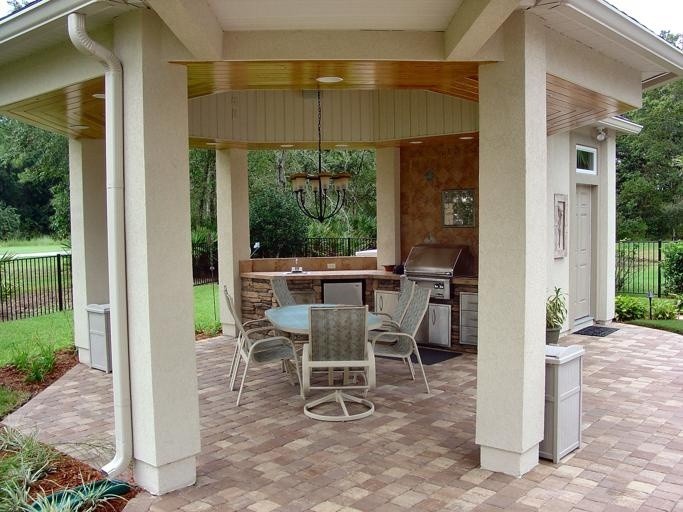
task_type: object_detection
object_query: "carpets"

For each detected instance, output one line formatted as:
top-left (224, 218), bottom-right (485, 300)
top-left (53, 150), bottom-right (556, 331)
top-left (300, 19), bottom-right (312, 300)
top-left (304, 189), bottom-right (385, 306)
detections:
top-left (573, 325), bottom-right (619, 338)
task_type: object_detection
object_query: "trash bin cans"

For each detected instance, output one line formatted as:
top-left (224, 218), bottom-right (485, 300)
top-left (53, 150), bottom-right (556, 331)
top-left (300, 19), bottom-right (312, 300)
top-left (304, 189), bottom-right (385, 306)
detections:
top-left (86, 304), bottom-right (112, 374)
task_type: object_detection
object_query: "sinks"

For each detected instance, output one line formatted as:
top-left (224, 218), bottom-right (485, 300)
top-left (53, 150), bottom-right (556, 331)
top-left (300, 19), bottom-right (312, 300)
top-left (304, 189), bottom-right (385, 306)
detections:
top-left (286, 272), bottom-right (309, 275)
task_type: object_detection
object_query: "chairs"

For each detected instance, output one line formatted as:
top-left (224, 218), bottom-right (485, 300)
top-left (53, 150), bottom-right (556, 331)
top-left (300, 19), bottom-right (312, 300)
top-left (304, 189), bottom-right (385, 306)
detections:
top-left (222, 276), bottom-right (431, 421)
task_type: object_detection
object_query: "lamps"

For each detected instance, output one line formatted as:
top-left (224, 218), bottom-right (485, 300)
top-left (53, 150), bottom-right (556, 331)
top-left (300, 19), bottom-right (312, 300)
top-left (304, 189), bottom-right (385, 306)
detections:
top-left (593, 126), bottom-right (607, 143)
top-left (288, 89), bottom-right (352, 223)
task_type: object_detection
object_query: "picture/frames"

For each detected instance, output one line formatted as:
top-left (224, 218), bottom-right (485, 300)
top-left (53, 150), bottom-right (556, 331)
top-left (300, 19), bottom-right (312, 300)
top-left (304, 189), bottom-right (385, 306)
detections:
top-left (552, 192), bottom-right (567, 259)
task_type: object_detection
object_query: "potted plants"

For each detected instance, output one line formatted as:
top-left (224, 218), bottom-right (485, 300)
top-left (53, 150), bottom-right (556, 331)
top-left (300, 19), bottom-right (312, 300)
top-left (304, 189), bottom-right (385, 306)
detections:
top-left (546, 286), bottom-right (567, 344)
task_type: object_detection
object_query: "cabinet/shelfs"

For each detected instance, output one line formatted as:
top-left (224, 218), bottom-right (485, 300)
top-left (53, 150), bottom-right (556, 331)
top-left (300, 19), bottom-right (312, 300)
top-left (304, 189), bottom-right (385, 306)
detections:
top-left (370, 279), bottom-right (477, 355)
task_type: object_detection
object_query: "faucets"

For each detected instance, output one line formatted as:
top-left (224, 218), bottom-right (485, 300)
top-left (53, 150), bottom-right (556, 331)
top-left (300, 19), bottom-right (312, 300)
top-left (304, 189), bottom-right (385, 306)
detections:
top-left (291, 258), bottom-right (303, 271)
